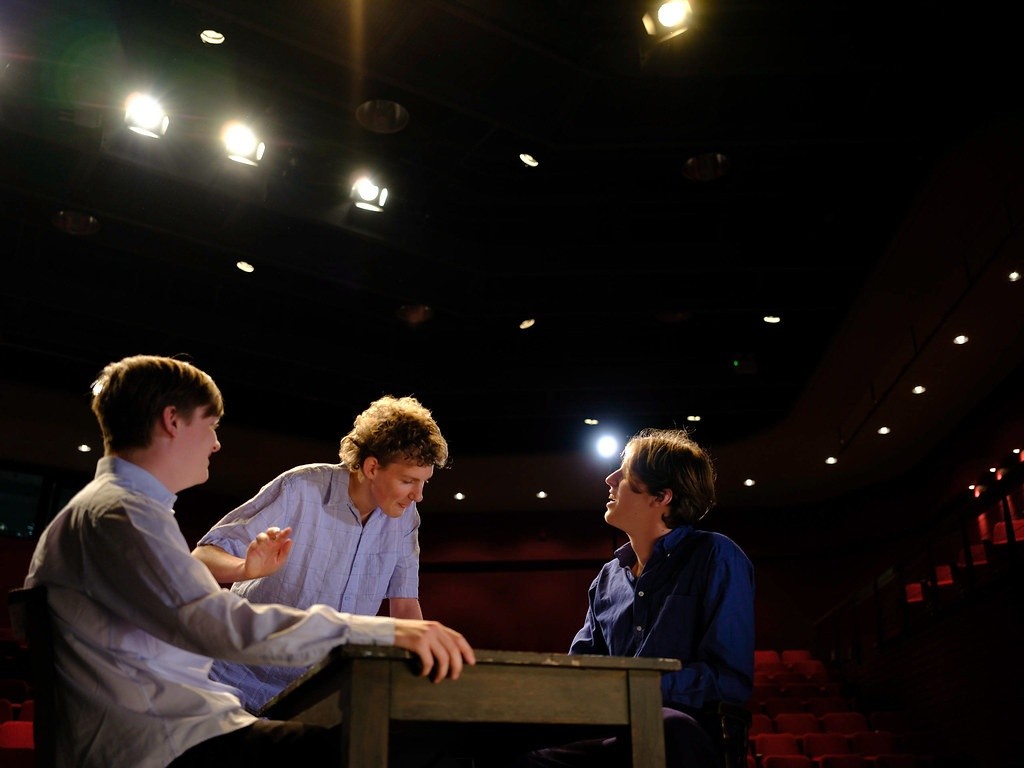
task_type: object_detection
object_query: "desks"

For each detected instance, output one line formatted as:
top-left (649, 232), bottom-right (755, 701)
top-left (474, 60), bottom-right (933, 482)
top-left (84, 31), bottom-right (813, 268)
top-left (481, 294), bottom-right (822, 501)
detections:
top-left (252, 641), bottom-right (682, 768)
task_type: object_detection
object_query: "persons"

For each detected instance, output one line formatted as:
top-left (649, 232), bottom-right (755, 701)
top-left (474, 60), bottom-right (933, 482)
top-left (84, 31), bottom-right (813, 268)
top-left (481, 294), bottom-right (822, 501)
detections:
top-left (567, 428), bottom-right (756, 768)
top-left (192, 397), bottom-right (449, 710)
top-left (24, 356), bottom-right (477, 768)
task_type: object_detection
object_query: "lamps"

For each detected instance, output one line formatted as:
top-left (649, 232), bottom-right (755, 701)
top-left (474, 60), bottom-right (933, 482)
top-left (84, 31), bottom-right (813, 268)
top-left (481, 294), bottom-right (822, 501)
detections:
top-left (125, 106), bottom-right (169, 139)
top-left (642, 0), bottom-right (694, 44)
top-left (349, 176), bottom-right (391, 213)
top-left (227, 134), bottom-right (266, 167)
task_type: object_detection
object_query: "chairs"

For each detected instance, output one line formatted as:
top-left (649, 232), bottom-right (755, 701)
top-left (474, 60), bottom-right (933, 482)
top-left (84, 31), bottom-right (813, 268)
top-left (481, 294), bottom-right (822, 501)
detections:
top-left (0, 628), bottom-right (36, 768)
top-left (748, 517), bottom-right (1024, 768)
